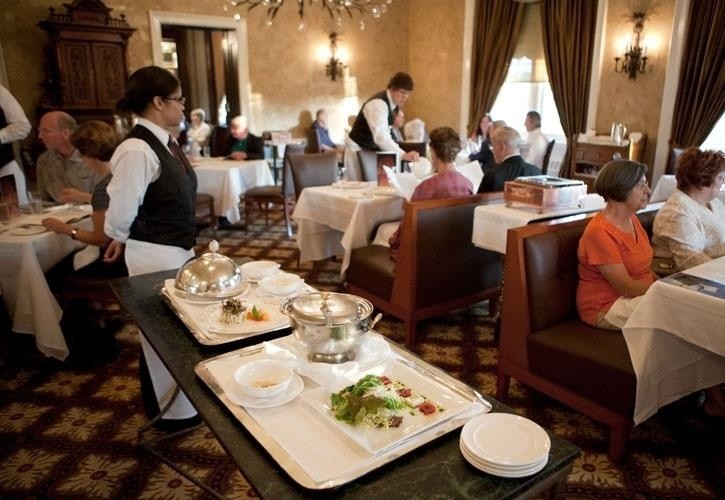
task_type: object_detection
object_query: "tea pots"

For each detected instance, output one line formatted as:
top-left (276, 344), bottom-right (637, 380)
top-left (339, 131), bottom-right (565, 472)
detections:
top-left (608, 121), bottom-right (627, 146)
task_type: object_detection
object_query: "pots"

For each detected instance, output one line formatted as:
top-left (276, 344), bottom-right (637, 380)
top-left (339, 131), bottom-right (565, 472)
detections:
top-left (278, 289), bottom-right (376, 366)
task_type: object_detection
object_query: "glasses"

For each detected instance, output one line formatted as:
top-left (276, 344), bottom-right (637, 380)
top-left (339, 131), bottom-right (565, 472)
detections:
top-left (158, 93), bottom-right (186, 106)
top-left (631, 180), bottom-right (652, 191)
top-left (488, 142), bottom-right (501, 151)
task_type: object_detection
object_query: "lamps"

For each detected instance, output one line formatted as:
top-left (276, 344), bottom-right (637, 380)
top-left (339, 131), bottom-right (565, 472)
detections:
top-left (223, 0), bottom-right (393, 33)
top-left (614, 11), bottom-right (650, 82)
top-left (321, 32), bottom-right (346, 80)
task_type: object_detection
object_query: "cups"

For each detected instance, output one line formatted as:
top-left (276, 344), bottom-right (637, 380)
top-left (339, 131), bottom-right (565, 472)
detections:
top-left (181, 143), bottom-right (210, 164)
top-left (0, 192), bottom-right (42, 226)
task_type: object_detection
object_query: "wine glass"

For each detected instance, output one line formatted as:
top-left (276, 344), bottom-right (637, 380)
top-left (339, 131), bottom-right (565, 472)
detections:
top-left (334, 166), bottom-right (347, 192)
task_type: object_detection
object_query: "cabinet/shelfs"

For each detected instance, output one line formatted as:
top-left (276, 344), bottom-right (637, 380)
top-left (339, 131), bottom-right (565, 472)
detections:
top-left (54, 37), bottom-right (127, 111)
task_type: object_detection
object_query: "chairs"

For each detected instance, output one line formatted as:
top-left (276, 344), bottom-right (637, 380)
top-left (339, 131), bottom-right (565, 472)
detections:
top-left (245, 144), bottom-right (302, 235)
top-left (305, 126), bottom-right (321, 154)
top-left (36, 280), bottom-right (143, 363)
top-left (287, 150), bottom-right (339, 262)
top-left (195, 194), bottom-right (217, 242)
top-left (357, 150), bottom-right (402, 181)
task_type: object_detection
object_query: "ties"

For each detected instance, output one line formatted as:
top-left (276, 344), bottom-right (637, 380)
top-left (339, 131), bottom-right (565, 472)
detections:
top-left (165, 134), bottom-right (191, 179)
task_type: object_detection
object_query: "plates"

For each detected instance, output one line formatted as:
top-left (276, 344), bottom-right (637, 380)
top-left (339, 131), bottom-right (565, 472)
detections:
top-left (173, 260), bottom-right (306, 335)
top-left (458, 411), bottom-right (552, 478)
top-left (7, 224), bottom-right (47, 236)
top-left (295, 357), bottom-right (473, 454)
top-left (330, 180), bottom-right (369, 189)
top-left (221, 373), bottom-right (304, 412)
top-left (364, 184), bottom-right (395, 197)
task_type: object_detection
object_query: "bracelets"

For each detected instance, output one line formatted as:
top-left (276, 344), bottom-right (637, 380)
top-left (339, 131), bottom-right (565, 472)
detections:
top-left (71, 226), bottom-right (80, 239)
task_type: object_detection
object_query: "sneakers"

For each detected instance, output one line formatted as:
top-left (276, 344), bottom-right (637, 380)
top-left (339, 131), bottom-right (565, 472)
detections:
top-left (699, 401), bottom-right (724, 419)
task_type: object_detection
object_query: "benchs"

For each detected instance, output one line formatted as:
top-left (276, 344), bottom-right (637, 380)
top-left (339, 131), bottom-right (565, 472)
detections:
top-left (495, 201), bottom-right (713, 462)
top-left (347, 190), bottom-right (504, 353)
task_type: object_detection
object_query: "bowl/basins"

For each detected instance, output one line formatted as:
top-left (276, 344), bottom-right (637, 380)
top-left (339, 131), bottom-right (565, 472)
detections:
top-left (235, 358), bottom-right (294, 397)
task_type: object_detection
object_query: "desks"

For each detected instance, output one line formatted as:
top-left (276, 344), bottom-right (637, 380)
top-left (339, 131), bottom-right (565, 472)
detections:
top-left (621, 256), bottom-right (724, 428)
top-left (290, 180), bottom-right (403, 275)
top-left (190, 157), bottom-right (276, 223)
top-left (1, 200), bottom-right (94, 361)
top-left (109, 256), bottom-right (581, 500)
top-left (571, 134), bottom-right (645, 194)
top-left (471, 193), bottom-right (607, 338)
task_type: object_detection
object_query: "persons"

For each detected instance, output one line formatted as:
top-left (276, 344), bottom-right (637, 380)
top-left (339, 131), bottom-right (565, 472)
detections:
top-left (344, 73), bottom-right (421, 181)
top-left (372, 125), bottom-right (474, 265)
top-left (39, 120), bottom-right (121, 335)
top-left (649, 150), bottom-right (724, 272)
top-left (576, 161), bottom-right (724, 420)
top-left (310, 110), bottom-right (340, 152)
top-left (34, 109), bottom-right (103, 207)
top-left (468, 110), bottom-right (549, 196)
top-left (102, 65), bottom-right (202, 433)
top-left (0, 81), bottom-right (31, 189)
top-left (177, 107), bottom-right (264, 160)
top-left (391, 110), bottom-right (406, 142)
